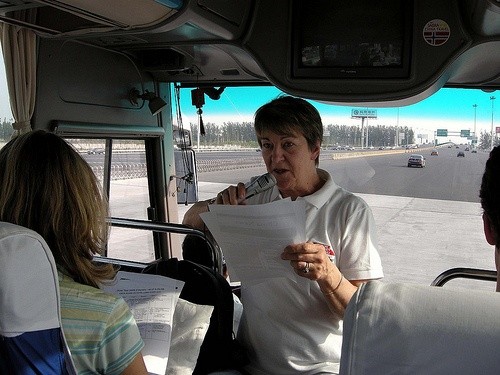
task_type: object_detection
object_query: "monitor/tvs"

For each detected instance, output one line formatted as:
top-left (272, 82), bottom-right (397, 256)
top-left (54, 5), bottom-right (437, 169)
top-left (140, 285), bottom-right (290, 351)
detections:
top-left (293, 0), bottom-right (411, 77)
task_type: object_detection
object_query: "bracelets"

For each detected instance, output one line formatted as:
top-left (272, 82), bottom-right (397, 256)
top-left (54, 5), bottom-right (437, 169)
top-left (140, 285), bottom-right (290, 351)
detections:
top-left (321, 274), bottom-right (343, 296)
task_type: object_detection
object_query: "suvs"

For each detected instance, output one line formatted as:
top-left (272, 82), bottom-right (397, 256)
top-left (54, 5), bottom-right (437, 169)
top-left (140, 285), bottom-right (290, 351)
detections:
top-left (408, 154), bottom-right (426, 168)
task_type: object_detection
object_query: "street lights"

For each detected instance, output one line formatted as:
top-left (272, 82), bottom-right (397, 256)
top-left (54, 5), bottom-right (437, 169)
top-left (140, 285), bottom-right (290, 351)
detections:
top-left (490, 95), bottom-right (496, 151)
top-left (473, 103), bottom-right (478, 140)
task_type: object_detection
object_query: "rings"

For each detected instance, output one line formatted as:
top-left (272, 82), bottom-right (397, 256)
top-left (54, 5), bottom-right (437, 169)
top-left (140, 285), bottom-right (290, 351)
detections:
top-left (303, 262), bottom-right (310, 273)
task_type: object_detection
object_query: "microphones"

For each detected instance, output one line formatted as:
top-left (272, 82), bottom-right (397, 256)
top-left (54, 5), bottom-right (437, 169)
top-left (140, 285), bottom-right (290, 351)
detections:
top-left (237, 173), bottom-right (277, 204)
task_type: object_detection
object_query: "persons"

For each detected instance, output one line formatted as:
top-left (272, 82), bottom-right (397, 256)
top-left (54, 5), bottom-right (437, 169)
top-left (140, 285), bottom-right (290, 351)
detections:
top-left (0, 129), bottom-right (147, 375)
top-left (181, 231), bottom-right (244, 339)
top-left (478, 143), bottom-right (500, 293)
top-left (181, 96), bottom-right (383, 375)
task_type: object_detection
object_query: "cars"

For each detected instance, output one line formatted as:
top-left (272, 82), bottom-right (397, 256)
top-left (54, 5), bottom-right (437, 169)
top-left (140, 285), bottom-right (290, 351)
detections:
top-left (256, 148), bottom-right (261, 152)
top-left (448, 145), bottom-right (452, 148)
top-left (465, 147), bottom-right (469, 151)
top-left (456, 146), bottom-right (459, 149)
top-left (87, 147), bottom-right (105, 154)
top-left (333, 145), bottom-right (355, 151)
top-left (431, 149), bottom-right (438, 155)
top-left (457, 151), bottom-right (465, 157)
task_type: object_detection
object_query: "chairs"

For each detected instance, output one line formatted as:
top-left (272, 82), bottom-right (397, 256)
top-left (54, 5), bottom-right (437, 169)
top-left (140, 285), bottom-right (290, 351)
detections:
top-left (0, 221), bottom-right (76, 375)
top-left (340, 280), bottom-right (500, 375)
